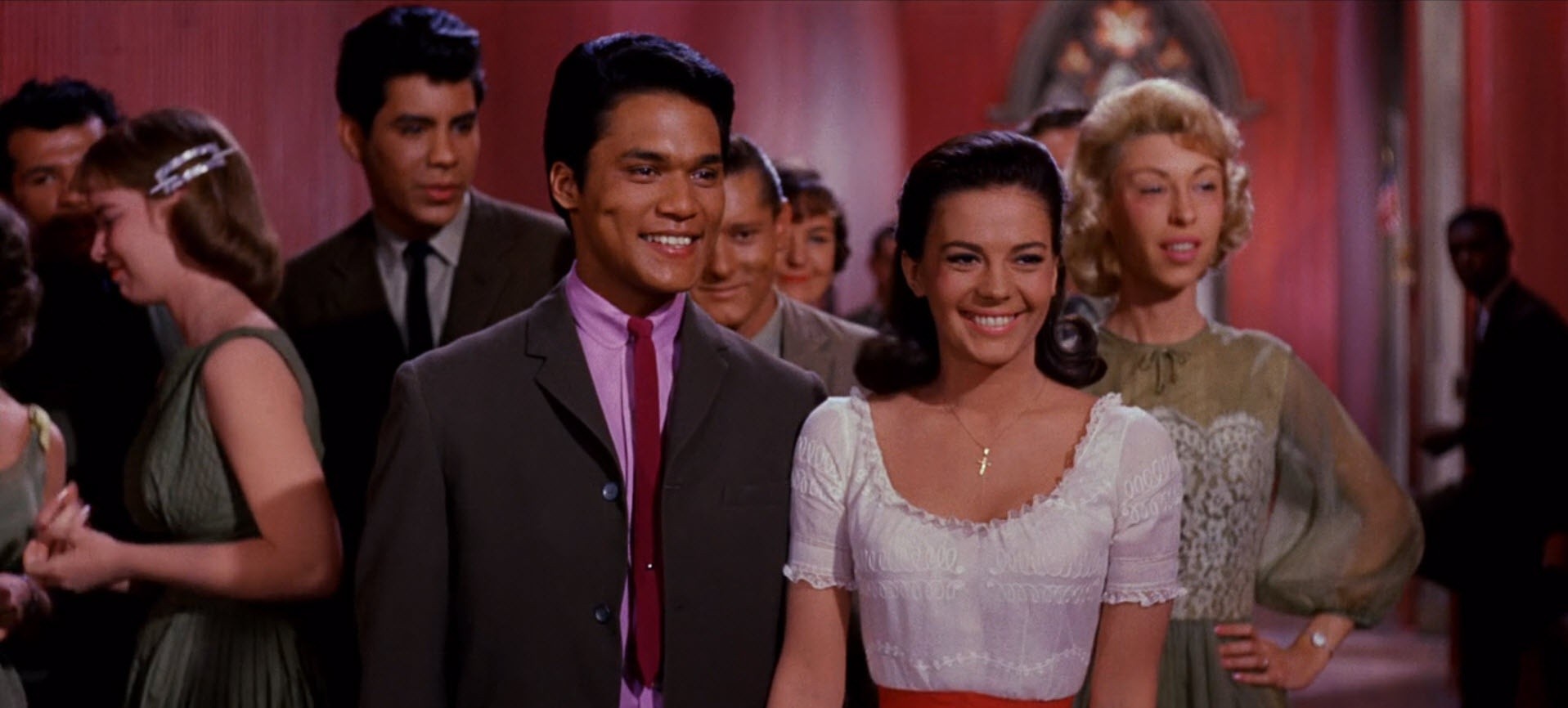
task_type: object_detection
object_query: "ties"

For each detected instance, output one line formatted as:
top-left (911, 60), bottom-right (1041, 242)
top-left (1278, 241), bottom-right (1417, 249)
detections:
top-left (624, 315), bottom-right (661, 687)
top-left (402, 240), bottom-right (433, 359)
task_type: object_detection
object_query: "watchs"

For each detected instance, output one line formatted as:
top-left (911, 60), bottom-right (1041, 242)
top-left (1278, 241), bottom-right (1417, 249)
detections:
top-left (1305, 627), bottom-right (1333, 655)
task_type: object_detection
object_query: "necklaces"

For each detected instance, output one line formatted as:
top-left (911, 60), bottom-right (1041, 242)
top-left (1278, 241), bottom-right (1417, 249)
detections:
top-left (944, 376), bottom-right (1048, 476)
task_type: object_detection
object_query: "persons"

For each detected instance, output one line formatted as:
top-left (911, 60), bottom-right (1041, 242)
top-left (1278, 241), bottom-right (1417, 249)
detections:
top-left (774, 162), bottom-right (852, 302)
top-left (1018, 105), bottom-right (1090, 295)
top-left (354, 31), bottom-right (829, 708)
top-left (766, 130), bottom-right (1189, 708)
top-left (0, 199), bottom-right (66, 708)
top-left (1421, 204), bottom-right (1568, 708)
top-left (846, 224), bottom-right (897, 332)
top-left (22, 102), bottom-right (345, 708)
top-left (690, 136), bottom-right (879, 396)
top-left (0, 75), bottom-right (185, 508)
top-left (1063, 76), bottom-right (1425, 708)
top-left (271, 4), bottom-right (577, 537)
